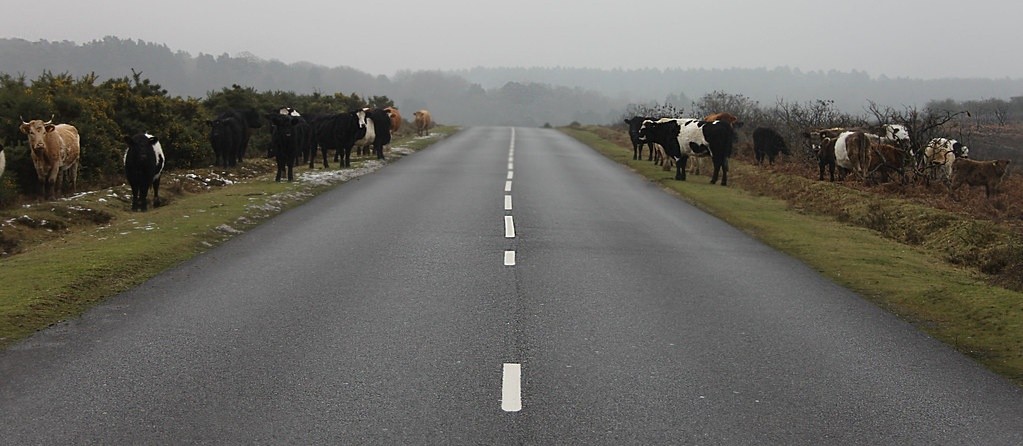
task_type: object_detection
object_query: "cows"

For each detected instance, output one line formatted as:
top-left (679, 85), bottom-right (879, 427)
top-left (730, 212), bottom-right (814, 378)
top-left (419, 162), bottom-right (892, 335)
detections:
top-left (623, 112), bottom-right (1012, 199)
top-left (0, 104), bottom-right (432, 212)
